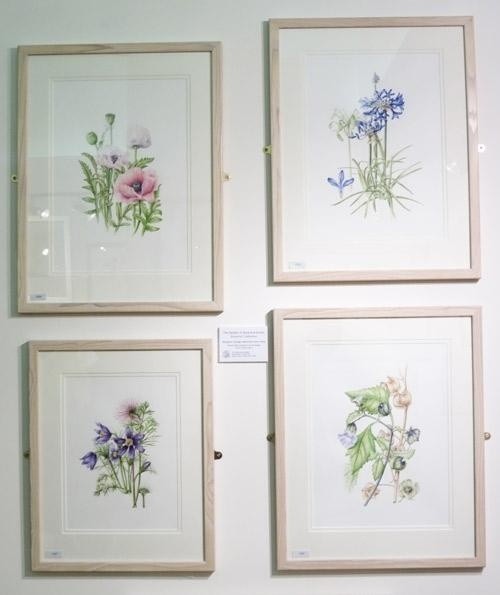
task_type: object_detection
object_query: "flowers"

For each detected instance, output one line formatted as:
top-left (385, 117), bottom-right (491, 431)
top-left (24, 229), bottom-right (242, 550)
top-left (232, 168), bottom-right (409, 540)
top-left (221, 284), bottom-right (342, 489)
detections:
top-left (324, 71), bottom-right (423, 222)
top-left (79, 399), bottom-right (163, 509)
top-left (77, 113), bottom-right (164, 236)
top-left (336, 364), bottom-right (422, 507)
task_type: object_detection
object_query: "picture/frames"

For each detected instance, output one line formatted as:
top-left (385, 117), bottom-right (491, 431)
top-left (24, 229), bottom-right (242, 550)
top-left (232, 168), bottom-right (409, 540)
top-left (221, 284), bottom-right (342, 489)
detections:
top-left (272, 307), bottom-right (486, 573)
top-left (15, 40), bottom-right (222, 314)
top-left (28, 339), bottom-right (215, 574)
top-left (267, 15), bottom-right (481, 285)
